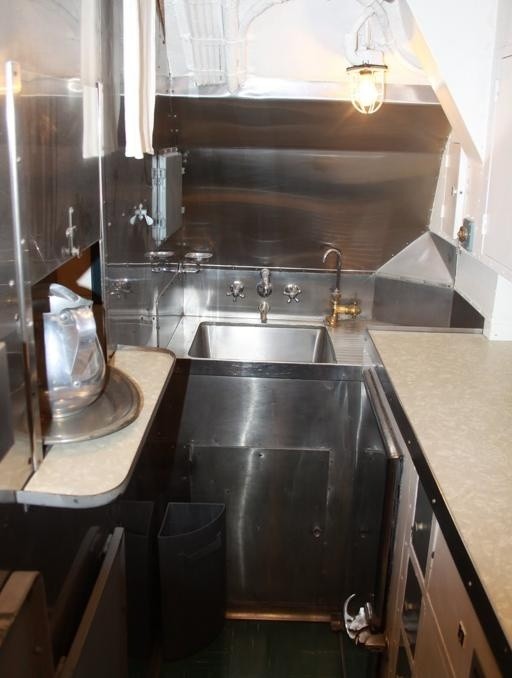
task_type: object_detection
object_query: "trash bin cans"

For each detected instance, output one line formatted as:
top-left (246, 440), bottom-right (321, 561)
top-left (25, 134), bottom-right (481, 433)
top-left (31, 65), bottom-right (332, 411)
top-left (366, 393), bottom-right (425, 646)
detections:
top-left (158, 502), bottom-right (226, 577)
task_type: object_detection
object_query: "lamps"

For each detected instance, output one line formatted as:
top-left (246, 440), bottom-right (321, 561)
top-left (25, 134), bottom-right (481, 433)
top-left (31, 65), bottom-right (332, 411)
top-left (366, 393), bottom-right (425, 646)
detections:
top-left (340, 1), bottom-right (392, 116)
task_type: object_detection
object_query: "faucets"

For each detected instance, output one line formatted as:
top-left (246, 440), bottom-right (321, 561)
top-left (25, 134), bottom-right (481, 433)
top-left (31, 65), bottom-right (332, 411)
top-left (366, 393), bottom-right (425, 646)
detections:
top-left (321, 249), bottom-right (360, 326)
top-left (258, 301), bottom-right (271, 323)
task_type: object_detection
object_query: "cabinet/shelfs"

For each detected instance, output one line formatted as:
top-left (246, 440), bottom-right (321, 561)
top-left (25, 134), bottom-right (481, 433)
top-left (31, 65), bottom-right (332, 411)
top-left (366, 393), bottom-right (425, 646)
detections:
top-left (379, 464), bottom-right (508, 677)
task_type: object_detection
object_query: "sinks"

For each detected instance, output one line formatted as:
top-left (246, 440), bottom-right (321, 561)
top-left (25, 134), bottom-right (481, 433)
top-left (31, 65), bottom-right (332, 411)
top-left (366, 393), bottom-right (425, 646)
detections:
top-left (186, 319), bottom-right (340, 366)
top-left (105, 311), bottom-right (183, 349)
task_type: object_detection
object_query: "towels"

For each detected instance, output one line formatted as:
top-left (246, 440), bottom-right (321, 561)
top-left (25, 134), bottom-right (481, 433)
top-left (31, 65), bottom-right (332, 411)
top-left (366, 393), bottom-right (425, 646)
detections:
top-left (124, 0), bottom-right (156, 160)
top-left (79, 2), bottom-right (120, 164)
